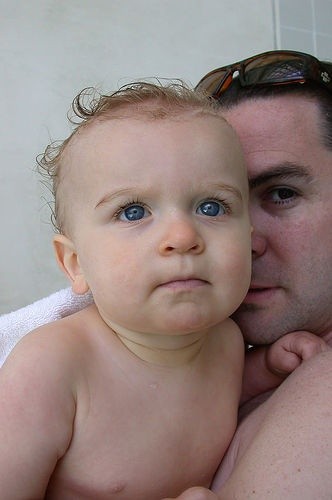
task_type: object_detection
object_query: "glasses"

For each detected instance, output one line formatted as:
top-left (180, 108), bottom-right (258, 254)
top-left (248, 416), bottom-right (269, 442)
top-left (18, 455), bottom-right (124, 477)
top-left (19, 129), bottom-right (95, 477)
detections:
top-left (194, 50), bottom-right (332, 101)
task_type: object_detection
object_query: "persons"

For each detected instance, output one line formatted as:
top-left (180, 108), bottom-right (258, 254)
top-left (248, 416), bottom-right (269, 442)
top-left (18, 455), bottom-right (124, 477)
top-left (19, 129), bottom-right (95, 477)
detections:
top-left (0, 78), bottom-right (324, 500)
top-left (194, 49), bottom-right (332, 500)
